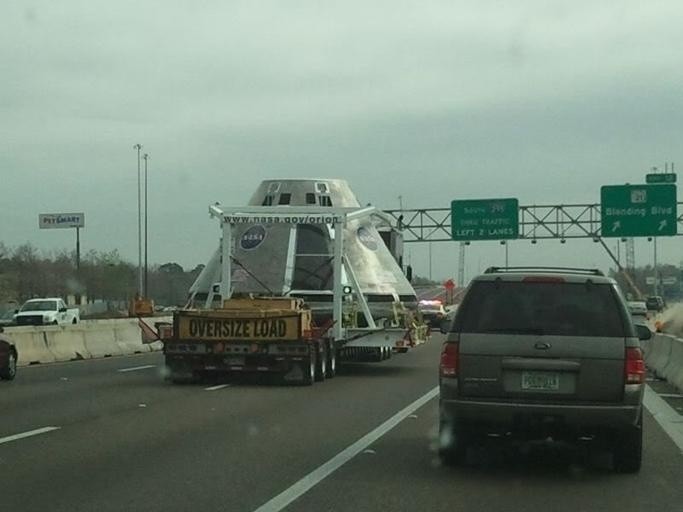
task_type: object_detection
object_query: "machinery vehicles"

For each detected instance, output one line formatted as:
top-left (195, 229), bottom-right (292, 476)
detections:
top-left (163, 178), bottom-right (426, 385)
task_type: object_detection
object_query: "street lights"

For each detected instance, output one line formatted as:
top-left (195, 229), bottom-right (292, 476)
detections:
top-left (132, 142), bottom-right (148, 299)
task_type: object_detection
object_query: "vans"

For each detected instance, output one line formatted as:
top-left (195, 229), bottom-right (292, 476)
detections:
top-left (644, 296), bottom-right (665, 313)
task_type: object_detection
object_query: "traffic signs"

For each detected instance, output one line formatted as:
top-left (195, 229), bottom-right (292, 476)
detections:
top-left (451, 199), bottom-right (518, 240)
top-left (602, 185), bottom-right (677, 239)
top-left (646, 173), bottom-right (677, 184)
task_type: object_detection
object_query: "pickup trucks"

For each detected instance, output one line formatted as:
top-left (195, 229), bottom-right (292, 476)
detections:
top-left (12, 298), bottom-right (79, 326)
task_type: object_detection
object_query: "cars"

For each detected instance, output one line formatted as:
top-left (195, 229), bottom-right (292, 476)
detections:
top-left (154, 304), bottom-right (177, 313)
top-left (628, 300), bottom-right (647, 317)
top-left (415, 298), bottom-right (449, 328)
top-left (0, 325), bottom-right (18, 381)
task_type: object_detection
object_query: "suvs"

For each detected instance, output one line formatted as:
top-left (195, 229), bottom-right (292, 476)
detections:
top-left (436, 266), bottom-right (653, 474)
